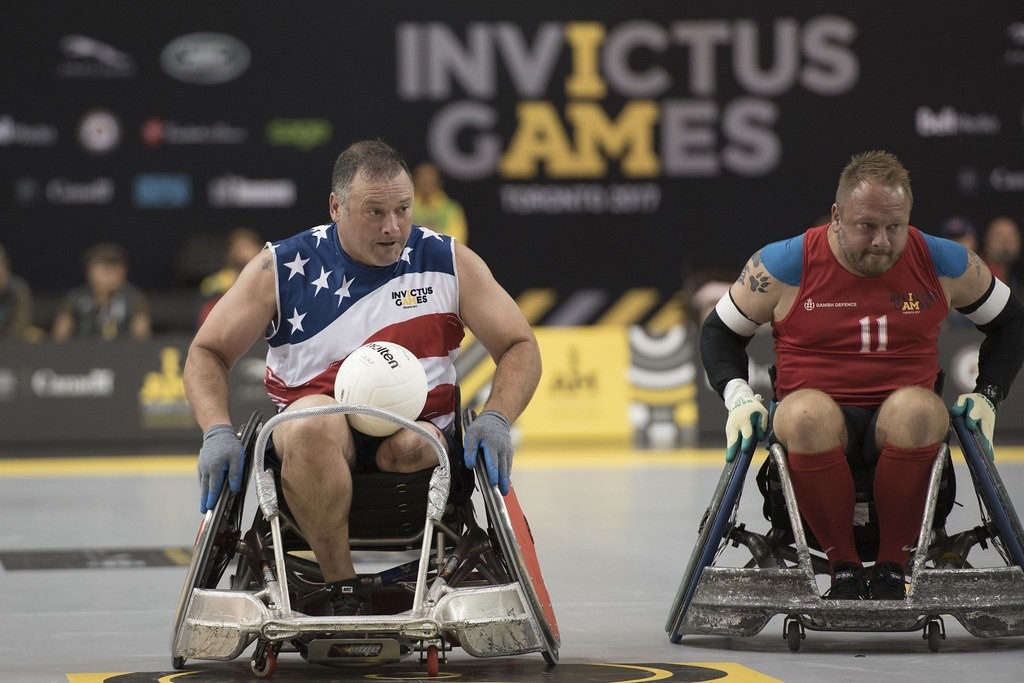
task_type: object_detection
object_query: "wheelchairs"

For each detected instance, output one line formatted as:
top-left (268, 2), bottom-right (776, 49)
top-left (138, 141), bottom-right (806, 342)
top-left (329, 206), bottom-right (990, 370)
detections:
top-left (171, 383), bottom-right (562, 678)
top-left (666, 364), bottom-right (1024, 651)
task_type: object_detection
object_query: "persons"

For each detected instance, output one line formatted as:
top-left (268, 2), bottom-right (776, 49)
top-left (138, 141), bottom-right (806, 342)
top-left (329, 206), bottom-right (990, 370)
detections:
top-left (0, 244), bottom-right (150, 343)
top-left (185, 141), bottom-right (542, 617)
top-left (701, 150), bottom-right (1024, 600)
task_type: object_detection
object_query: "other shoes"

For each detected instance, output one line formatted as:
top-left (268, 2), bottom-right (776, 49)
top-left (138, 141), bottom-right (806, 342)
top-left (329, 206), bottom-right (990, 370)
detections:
top-left (325, 576), bottom-right (366, 637)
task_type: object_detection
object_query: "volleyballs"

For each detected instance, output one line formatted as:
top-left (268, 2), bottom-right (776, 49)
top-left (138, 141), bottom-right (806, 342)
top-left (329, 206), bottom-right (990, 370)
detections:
top-left (332, 341), bottom-right (430, 438)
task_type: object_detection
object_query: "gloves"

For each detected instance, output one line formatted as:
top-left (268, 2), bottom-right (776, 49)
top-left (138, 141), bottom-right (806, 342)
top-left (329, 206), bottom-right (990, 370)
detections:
top-left (464, 410), bottom-right (514, 496)
top-left (197, 424), bottom-right (245, 514)
top-left (724, 394), bottom-right (769, 463)
top-left (951, 392), bottom-right (997, 463)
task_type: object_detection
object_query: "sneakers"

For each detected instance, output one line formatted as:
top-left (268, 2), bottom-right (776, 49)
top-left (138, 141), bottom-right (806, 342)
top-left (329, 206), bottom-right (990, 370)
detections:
top-left (869, 561), bottom-right (906, 599)
top-left (820, 561), bottom-right (868, 600)
